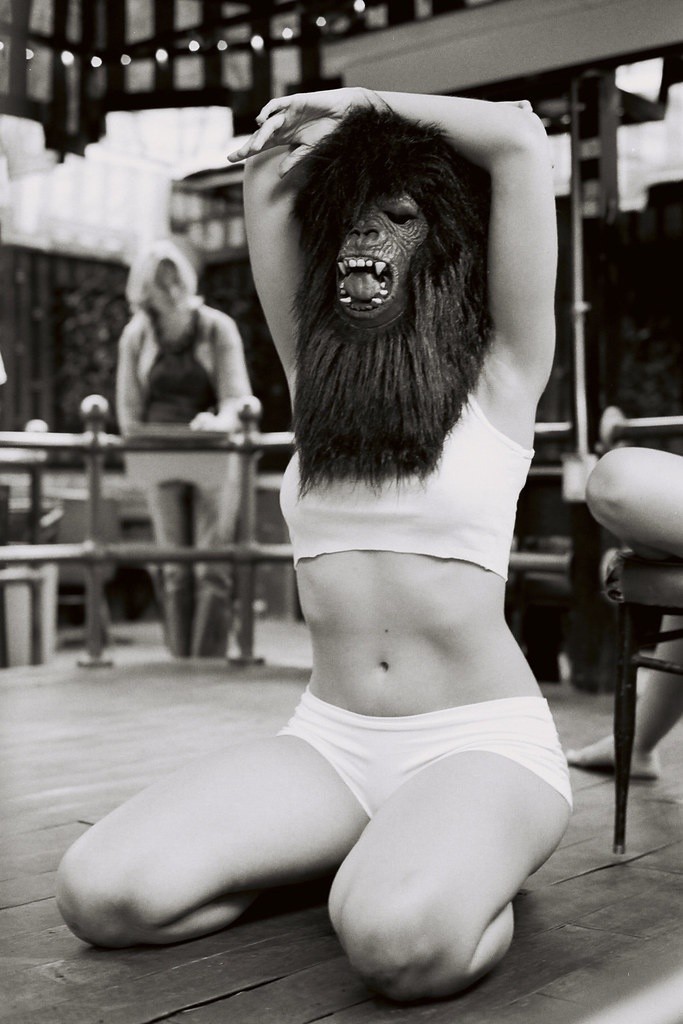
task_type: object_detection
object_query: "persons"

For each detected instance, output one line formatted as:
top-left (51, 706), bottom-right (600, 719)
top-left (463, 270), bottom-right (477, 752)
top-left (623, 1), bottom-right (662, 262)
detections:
top-left (566, 446), bottom-right (683, 779)
top-left (52, 87), bottom-right (574, 1001)
top-left (118, 242), bottom-right (250, 661)
